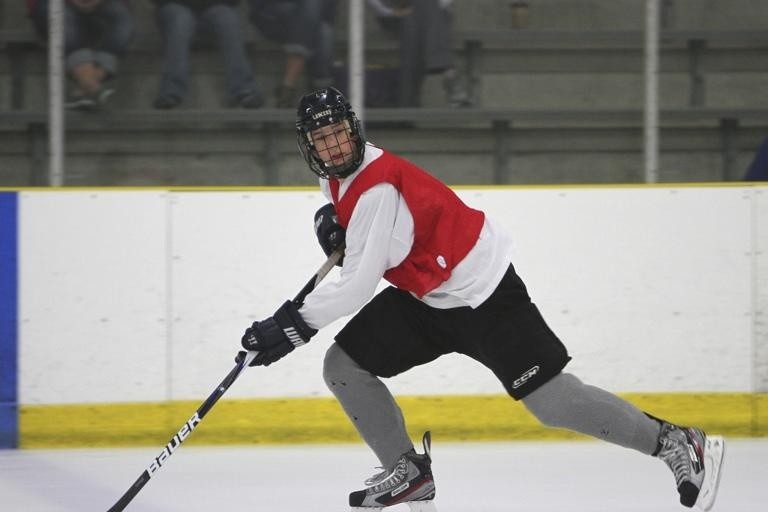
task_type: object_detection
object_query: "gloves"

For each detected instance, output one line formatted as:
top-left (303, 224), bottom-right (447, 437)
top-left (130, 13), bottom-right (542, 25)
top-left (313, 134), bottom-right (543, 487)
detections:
top-left (314, 203), bottom-right (346, 267)
top-left (235, 300), bottom-right (319, 366)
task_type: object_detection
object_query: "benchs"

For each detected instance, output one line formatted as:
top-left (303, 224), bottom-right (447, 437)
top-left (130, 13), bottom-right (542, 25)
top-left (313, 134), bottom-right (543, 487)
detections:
top-left (0, 1), bottom-right (768, 188)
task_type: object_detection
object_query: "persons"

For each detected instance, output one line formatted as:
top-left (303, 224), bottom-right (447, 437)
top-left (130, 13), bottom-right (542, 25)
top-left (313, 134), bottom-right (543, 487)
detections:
top-left (234, 86), bottom-right (711, 507)
top-left (29, 1), bottom-right (481, 110)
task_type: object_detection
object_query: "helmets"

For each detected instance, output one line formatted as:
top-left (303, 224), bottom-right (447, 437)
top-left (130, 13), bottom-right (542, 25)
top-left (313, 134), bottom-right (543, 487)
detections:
top-left (295, 87), bottom-right (365, 180)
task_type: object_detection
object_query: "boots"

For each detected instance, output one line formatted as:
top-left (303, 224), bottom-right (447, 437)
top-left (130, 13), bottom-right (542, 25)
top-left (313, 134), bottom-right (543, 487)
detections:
top-left (643, 411), bottom-right (705, 506)
top-left (348, 431), bottom-right (436, 506)
